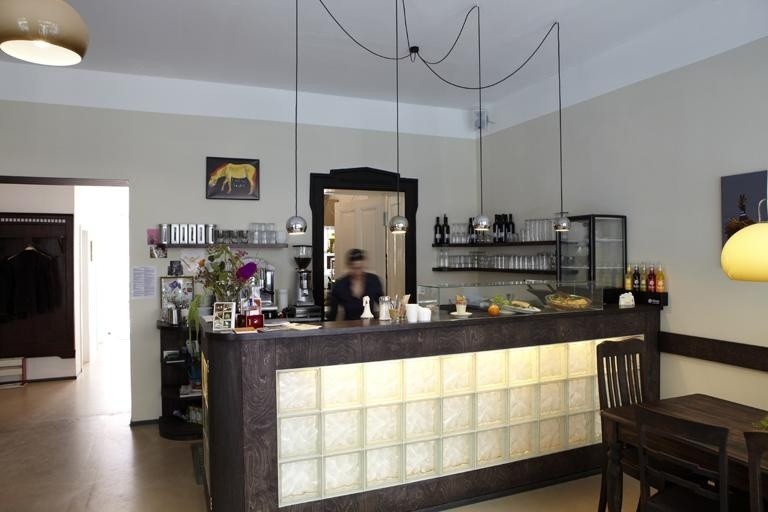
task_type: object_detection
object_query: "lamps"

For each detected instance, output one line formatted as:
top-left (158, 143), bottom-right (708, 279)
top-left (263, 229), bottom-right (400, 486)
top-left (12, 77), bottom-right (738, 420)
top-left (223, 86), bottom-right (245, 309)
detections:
top-left (719, 198), bottom-right (768, 285)
top-left (286, 0), bottom-right (572, 238)
top-left (0, 1), bottom-right (91, 68)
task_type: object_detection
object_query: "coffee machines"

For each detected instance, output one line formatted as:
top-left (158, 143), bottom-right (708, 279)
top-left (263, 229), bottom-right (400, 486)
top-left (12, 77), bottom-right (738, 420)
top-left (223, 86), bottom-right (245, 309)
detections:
top-left (260, 268), bottom-right (278, 319)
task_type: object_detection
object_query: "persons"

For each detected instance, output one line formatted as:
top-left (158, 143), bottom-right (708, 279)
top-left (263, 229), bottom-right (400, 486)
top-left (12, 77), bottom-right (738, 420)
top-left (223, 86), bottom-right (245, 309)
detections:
top-left (329, 249), bottom-right (384, 321)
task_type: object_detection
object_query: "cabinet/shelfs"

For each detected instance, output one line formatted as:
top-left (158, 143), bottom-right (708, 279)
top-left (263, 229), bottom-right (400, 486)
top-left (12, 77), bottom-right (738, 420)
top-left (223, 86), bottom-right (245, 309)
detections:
top-left (554, 212), bottom-right (628, 289)
top-left (156, 318), bottom-right (203, 442)
top-left (429, 238), bottom-right (577, 277)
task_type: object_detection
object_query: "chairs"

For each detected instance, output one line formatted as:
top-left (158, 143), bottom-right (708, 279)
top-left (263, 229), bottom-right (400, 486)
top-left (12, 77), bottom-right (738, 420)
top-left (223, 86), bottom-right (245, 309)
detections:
top-left (738, 429), bottom-right (767, 512)
top-left (597, 338), bottom-right (708, 510)
top-left (631, 398), bottom-right (732, 510)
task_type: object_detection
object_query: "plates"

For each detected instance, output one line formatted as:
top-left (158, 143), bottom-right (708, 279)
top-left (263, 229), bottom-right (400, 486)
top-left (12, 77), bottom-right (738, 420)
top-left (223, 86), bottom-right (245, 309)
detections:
top-left (449, 312), bottom-right (472, 319)
top-left (504, 304), bottom-right (541, 313)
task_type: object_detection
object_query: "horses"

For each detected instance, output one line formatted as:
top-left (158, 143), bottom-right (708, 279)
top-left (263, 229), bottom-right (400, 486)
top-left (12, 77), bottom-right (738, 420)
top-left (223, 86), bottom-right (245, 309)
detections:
top-left (208, 163), bottom-right (259, 199)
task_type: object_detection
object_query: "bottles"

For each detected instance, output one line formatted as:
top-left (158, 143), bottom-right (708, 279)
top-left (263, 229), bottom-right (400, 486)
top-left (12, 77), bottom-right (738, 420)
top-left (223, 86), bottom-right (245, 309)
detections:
top-left (159, 224), bottom-right (170, 245)
top-left (166, 306), bottom-right (182, 326)
top-left (624, 263), bottom-right (666, 293)
top-left (217, 223), bottom-right (276, 244)
top-left (205, 224), bottom-right (216, 245)
top-left (378, 296), bottom-right (391, 321)
top-left (432, 212), bottom-right (571, 273)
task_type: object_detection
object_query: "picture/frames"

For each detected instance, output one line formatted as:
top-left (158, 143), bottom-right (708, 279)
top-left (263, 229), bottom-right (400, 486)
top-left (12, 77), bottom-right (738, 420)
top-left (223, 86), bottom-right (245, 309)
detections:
top-left (160, 276), bottom-right (195, 317)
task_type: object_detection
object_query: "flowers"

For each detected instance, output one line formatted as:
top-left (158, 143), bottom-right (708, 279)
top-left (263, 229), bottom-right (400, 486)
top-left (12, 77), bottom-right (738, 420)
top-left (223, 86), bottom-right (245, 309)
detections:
top-left (179, 239), bottom-right (258, 357)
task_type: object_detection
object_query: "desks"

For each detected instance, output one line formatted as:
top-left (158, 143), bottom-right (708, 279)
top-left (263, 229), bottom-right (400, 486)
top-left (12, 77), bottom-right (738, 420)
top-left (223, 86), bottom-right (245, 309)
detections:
top-left (598, 391), bottom-right (767, 512)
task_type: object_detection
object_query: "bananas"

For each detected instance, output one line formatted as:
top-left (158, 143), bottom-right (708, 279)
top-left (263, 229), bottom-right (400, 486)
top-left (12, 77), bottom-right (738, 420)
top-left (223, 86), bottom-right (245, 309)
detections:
top-left (512, 300), bottom-right (530, 308)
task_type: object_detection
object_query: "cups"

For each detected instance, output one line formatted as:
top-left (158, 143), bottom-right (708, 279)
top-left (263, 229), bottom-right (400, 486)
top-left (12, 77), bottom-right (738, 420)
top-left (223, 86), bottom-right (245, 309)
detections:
top-left (276, 289), bottom-right (288, 318)
top-left (390, 292), bottom-right (406, 322)
top-left (405, 304), bottom-right (431, 324)
top-left (455, 304), bottom-right (466, 313)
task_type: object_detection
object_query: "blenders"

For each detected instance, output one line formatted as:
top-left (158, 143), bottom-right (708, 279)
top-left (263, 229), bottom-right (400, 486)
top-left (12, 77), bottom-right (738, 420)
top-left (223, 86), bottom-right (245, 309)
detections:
top-left (291, 245), bottom-right (314, 307)
